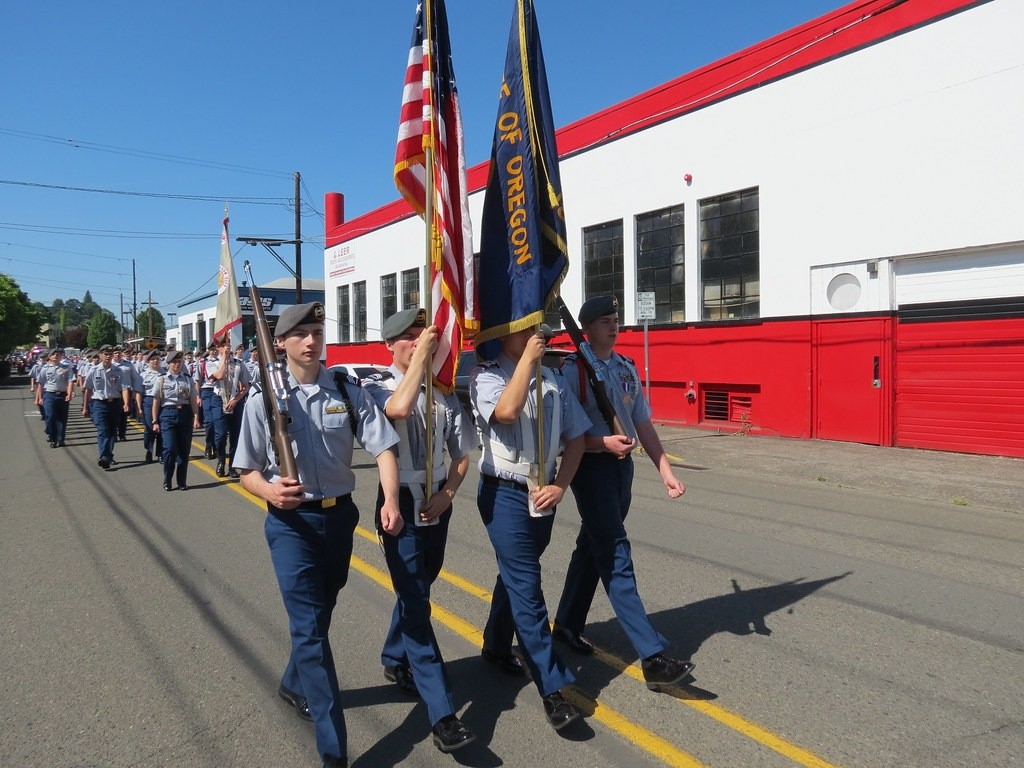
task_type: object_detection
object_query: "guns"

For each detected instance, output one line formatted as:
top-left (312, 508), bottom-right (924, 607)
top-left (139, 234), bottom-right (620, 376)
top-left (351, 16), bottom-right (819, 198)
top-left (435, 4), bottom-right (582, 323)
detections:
top-left (552, 292), bottom-right (632, 446)
top-left (242, 257), bottom-right (305, 499)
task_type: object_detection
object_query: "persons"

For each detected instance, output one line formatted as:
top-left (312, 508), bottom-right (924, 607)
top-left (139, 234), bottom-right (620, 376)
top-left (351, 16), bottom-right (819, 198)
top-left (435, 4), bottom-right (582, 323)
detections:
top-left (552, 294), bottom-right (696, 690)
top-left (469, 326), bottom-right (594, 730)
top-left (361, 309), bottom-right (476, 751)
top-left (7, 335), bottom-right (261, 491)
top-left (232, 302), bottom-right (404, 768)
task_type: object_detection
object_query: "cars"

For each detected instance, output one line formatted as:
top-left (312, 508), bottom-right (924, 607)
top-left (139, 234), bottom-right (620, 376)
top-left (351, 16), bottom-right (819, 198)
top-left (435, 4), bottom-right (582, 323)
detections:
top-left (326, 363), bottom-right (389, 382)
top-left (9, 352), bottom-right (23, 362)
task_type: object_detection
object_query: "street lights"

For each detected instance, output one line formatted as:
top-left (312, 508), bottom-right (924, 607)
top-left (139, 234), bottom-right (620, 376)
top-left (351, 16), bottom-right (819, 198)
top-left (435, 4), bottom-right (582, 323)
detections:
top-left (167, 313), bottom-right (176, 326)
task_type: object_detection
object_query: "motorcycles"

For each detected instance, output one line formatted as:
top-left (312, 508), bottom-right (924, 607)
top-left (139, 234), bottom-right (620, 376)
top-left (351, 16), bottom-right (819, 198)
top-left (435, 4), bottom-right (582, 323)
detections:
top-left (17, 365), bottom-right (25, 375)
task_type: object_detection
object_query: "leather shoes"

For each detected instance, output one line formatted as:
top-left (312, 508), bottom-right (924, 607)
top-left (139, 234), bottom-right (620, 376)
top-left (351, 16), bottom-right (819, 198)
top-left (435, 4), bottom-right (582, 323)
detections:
top-left (641, 653), bottom-right (696, 689)
top-left (542, 691), bottom-right (580, 730)
top-left (482, 643), bottom-right (528, 677)
top-left (384, 665), bottom-right (420, 696)
top-left (279, 684), bottom-right (313, 721)
top-left (323, 757), bottom-right (348, 768)
top-left (433, 715), bottom-right (476, 750)
top-left (551, 622), bottom-right (594, 655)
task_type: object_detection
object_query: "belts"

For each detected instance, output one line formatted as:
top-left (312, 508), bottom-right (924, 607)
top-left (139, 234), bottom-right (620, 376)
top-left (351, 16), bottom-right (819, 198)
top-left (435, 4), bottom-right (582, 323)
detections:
top-left (94, 398), bottom-right (121, 402)
top-left (49, 391), bottom-right (66, 395)
top-left (267, 493), bottom-right (351, 508)
top-left (165, 404), bottom-right (190, 409)
top-left (479, 472), bottom-right (529, 492)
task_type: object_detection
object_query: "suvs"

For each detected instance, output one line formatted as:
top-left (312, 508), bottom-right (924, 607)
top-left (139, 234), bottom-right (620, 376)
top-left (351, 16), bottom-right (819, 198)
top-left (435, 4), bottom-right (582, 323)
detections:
top-left (453, 349), bottom-right (575, 425)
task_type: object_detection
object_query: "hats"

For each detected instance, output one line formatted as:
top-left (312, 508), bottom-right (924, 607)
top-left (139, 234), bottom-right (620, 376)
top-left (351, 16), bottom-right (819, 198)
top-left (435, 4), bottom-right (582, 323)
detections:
top-left (39, 348), bottom-right (65, 357)
top-left (381, 308), bottom-right (426, 339)
top-left (275, 301), bottom-right (325, 336)
top-left (578, 296), bottom-right (618, 322)
top-left (82, 342), bottom-right (258, 364)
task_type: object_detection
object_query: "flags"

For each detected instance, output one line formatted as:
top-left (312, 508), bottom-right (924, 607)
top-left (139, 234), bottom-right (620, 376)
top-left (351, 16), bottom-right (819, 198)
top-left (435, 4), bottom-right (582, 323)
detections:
top-left (472, 0), bottom-right (570, 368)
top-left (214, 218), bottom-right (243, 343)
top-left (393, 0), bottom-right (480, 396)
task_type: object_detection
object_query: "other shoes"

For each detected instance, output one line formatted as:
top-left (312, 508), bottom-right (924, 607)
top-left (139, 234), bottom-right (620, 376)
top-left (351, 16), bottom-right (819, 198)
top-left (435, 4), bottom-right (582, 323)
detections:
top-left (157, 456), bottom-right (164, 464)
top-left (216, 462), bottom-right (224, 477)
top-left (146, 451), bottom-right (152, 462)
top-left (227, 468), bottom-right (239, 478)
top-left (177, 484), bottom-right (187, 490)
top-left (50, 441), bottom-right (65, 448)
top-left (203, 449), bottom-right (218, 460)
top-left (163, 482), bottom-right (172, 491)
top-left (98, 459), bottom-right (116, 468)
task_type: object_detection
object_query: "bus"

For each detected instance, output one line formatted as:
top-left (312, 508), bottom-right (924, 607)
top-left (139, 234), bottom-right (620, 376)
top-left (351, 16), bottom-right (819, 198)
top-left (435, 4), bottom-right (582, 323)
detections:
top-left (59, 346), bottom-right (80, 358)
